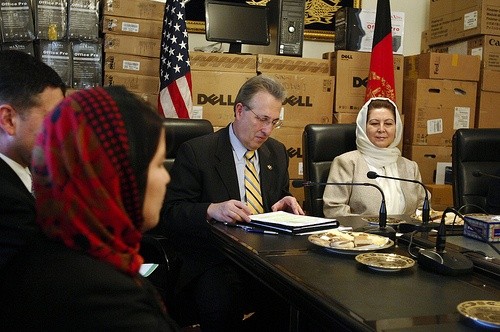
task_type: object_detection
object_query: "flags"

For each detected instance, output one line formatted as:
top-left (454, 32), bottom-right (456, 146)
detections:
top-left (364, 0), bottom-right (396, 104)
top-left (157, 0), bottom-right (193, 119)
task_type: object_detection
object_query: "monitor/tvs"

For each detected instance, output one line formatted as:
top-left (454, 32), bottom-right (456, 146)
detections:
top-left (205, 0), bottom-right (270, 54)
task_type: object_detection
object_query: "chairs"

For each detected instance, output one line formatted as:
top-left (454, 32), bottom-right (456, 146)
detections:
top-left (451, 127), bottom-right (500, 216)
top-left (302, 123), bottom-right (358, 219)
top-left (136, 118), bottom-right (215, 332)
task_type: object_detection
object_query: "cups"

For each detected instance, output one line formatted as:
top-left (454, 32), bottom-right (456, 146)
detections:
top-left (415, 209), bottom-right (433, 216)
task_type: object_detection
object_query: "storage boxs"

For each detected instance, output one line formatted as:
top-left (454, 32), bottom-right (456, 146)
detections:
top-left (0, 0), bottom-right (500, 213)
top-left (463, 215), bottom-right (500, 243)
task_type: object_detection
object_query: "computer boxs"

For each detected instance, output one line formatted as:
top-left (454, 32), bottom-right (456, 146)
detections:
top-left (276, 0), bottom-right (305, 56)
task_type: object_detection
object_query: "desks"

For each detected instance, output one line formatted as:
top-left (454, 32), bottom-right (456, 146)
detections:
top-left (206, 211), bottom-right (500, 332)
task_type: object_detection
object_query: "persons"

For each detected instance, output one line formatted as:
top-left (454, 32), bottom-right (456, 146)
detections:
top-left (0, 50), bottom-right (67, 332)
top-left (323, 96), bottom-right (431, 217)
top-left (158, 77), bottom-right (306, 332)
top-left (0, 84), bottom-right (175, 332)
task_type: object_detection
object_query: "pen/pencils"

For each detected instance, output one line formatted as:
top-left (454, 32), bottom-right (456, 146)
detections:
top-left (224, 223), bottom-right (255, 229)
top-left (243, 228), bottom-right (279, 234)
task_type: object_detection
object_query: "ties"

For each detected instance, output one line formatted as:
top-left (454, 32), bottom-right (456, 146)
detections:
top-left (244, 150), bottom-right (264, 216)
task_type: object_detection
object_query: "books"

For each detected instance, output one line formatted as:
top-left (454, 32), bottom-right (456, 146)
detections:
top-left (247, 211), bottom-right (340, 234)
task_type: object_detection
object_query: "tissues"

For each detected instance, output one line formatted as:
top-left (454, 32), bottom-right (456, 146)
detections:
top-left (464, 216), bottom-right (500, 242)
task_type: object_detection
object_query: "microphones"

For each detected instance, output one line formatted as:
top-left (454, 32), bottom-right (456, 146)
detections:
top-left (291, 179), bottom-right (399, 243)
top-left (473, 171), bottom-right (500, 181)
top-left (367, 170), bottom-right (433, 233)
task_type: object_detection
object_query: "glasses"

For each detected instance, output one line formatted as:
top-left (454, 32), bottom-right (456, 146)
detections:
top-left (245, 104), bottom-right (283, 129)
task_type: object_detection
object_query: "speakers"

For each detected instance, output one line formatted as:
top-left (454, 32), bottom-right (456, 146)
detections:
top-left (335, 6), bottom-right (365, 51)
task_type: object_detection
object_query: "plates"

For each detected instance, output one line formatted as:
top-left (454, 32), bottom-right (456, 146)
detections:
top-left (364, 215), bottom-right (407, 225)
top-left (308, 229), bottom-right (396, 255)
top-left (457, 299), bottom-right (500, 330)
top-left (354, 253), bottom-right (415, 272)
top-left (410, 212), bottom-right (488, 226)
